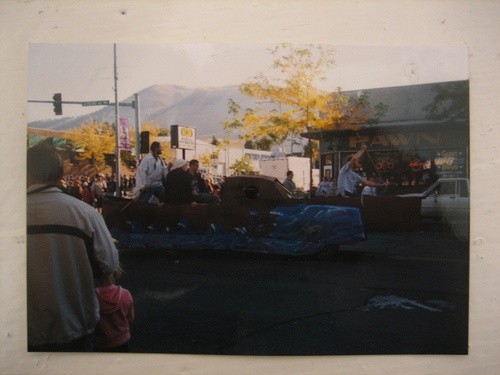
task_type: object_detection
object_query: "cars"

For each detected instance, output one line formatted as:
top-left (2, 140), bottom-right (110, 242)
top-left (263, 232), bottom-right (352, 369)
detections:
top-left (392, 177), bottom-right (469, 218)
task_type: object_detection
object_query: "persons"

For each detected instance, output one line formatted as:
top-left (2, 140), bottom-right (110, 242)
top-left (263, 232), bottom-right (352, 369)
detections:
top-left (58, 142), bottom-right (227, 213)
top-left (27, 142), bottom-right (119, 353)
top-left (282, 144), bottom-right (389, 197)
top-left (94, 266), bottom-right (135, 353)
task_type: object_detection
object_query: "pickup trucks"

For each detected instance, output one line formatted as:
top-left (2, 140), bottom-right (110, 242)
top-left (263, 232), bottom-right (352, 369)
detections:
top-left (102, 173), bottom-right (367, 262)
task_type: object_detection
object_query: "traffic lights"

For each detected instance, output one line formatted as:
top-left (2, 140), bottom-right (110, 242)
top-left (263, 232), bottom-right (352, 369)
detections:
top-left (52, 93), bottom-right (62, 115)
top-left (139, 130), bottom-right (150, 153)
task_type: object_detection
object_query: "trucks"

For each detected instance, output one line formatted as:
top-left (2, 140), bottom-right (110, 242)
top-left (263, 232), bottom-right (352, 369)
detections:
top-left (260, 156), bottom-right (321, 199)
